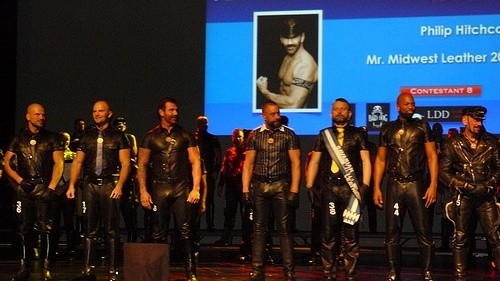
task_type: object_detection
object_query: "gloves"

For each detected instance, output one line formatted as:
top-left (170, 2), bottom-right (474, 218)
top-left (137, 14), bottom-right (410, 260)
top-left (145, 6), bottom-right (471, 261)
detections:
top-left (307, 186), bottom-right (321, 208)
top-left (286, 191), bottom-right (299, 209)
top-left (358, 183), bottom-right (369, 205)
top-left (19, 178), bottom-right (36, 193)
top-left (468, 182), bottom-right (487, 199)
top-left (36, 187), bottom-right (55, 202)
top-left (241, 191), bottom-right (253, 205)
top-left (452, 179), bottom-right (476, 193)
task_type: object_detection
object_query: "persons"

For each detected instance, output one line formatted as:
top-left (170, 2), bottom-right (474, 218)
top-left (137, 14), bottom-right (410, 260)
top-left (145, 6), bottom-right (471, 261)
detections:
top-left (306, 99), bottom-right (378, 281)
top-left (373, 93), bottom-right (439, 281)
top-left (137, 99), bottom-right (207, 281)
top-left (257, 16), bottom-right (317, 109)
top-left (242, 102), bottom-right (301, 281)
top-left (0, 103), bottom-right (85, 281)
top-left (66, 101), bottom-right (138, 281)
top-left (432, 123), bottom-right (476, 250)
top-left (439, 107), bottom-right (500, 281)
top-left (197, 115), bottom-right (299, 245)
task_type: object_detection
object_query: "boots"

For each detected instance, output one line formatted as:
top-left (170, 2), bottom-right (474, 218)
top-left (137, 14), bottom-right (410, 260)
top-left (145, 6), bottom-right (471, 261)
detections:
top-left (435, 217), bottom-right (452, 251)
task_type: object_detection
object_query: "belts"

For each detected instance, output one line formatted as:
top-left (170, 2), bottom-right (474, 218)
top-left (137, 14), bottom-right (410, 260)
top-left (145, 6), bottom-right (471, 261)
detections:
top-left (86, 177), bottom-right (119, 185)
top-left (254, 173), bottom-right (288, 183)
top-left (388, 172), bottom-right (423, 183)
top-left (31, 179), bottom-right (49, 184)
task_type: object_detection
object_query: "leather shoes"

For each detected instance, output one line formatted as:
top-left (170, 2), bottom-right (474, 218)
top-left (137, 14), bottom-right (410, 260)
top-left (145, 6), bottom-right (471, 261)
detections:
top-left (206, 224), bottom-right (218, 233)
top-left (236, 249), bottom-right (252, 262)
top-left (8, 258), bottom-right (30, 280)
top-left (40, 259), bottom-right (56, 280)
top-left (214, 238), bottom-right (231, 246)
top-left (263, 249), bottom-right (280, 263)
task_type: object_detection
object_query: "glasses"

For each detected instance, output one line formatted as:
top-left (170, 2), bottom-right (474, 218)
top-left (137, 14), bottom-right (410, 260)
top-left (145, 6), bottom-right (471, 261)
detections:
top-left (433, 126), bottom-right (442, 131)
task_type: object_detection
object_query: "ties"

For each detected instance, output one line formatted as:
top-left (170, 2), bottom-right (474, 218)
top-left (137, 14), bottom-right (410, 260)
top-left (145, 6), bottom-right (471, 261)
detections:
top-left (330, 127), bottom-right (345, 173)
top-left (95, 130), bottom-right (104, 175)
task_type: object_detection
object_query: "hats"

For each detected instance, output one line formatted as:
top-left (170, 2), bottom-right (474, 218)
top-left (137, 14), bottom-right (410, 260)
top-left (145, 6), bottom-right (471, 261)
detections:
top-left (462, 106), bottom-right (487, 120)
top-left (278, 19), bottom-right (304, 39)
top-left (113, 118), bottom-right (127, 125)
